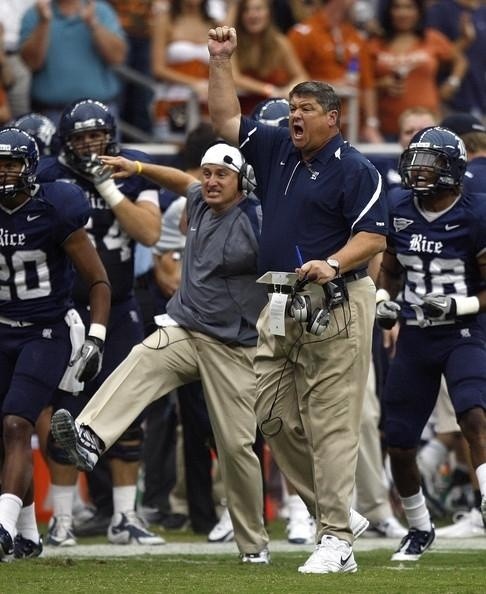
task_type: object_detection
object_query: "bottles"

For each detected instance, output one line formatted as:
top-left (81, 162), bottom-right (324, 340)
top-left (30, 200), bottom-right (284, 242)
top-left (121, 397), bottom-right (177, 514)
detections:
top-left (344, 57), bottom-right (360, 88)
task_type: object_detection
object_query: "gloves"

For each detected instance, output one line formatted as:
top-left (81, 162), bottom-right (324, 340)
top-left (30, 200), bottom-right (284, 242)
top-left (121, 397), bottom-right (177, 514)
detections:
top-left (68, 335), bottom-right (105, 383)
top-left (375, 300), bottom-right (401, 330)
top-left (422, 295), bottom-right (457, 321)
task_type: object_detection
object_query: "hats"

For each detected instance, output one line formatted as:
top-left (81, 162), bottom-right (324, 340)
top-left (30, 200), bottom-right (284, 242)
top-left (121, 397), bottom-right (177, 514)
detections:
top-left (442, 113), bottom-right (486, 138)
top-left (200, 143), bottom-right (247, 173)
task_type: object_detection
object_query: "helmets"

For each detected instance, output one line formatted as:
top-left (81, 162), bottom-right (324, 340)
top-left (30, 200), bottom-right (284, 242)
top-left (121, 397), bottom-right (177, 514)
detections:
top-left (249, 97), bottom-right (291, 127)
top-left (398, 127), bottom-right (468, 197)
top-left (1, 98), bottom-right (116, 206)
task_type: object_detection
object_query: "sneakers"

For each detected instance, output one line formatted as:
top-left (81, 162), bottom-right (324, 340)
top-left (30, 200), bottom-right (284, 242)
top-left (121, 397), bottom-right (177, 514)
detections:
top-left (207, 508), bottom-right (234, 542)
top-left (50, 408), bottom-right (103, 471)
top-left (433, 507), bottom-right (485, 538)
top-left (239, 548), bottom-right (270, 565)
top-left (287, 506), bottom-right (436, 575)
top-left (0, 507), bottom-right (191, 562)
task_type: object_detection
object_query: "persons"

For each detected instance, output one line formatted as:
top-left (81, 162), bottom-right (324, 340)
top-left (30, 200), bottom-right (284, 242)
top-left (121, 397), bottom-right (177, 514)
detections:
top-left (1, 0), bottom-right (485, 575)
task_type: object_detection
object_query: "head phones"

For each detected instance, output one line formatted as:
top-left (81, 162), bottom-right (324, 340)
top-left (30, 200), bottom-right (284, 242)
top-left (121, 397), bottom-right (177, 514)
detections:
top-left (201, 139), bottom-right (256, 195)
top-left (286, 277), bottom-right (335, 336)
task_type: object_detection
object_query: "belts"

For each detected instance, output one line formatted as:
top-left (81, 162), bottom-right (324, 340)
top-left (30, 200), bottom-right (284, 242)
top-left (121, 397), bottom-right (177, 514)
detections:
top-left (268, 269), bottom-right (368, 294)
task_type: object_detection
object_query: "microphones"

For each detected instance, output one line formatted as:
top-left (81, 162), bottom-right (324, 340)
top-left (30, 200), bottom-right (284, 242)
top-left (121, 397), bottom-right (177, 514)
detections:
top-left (223, 155), bottom-right (258, 188)
